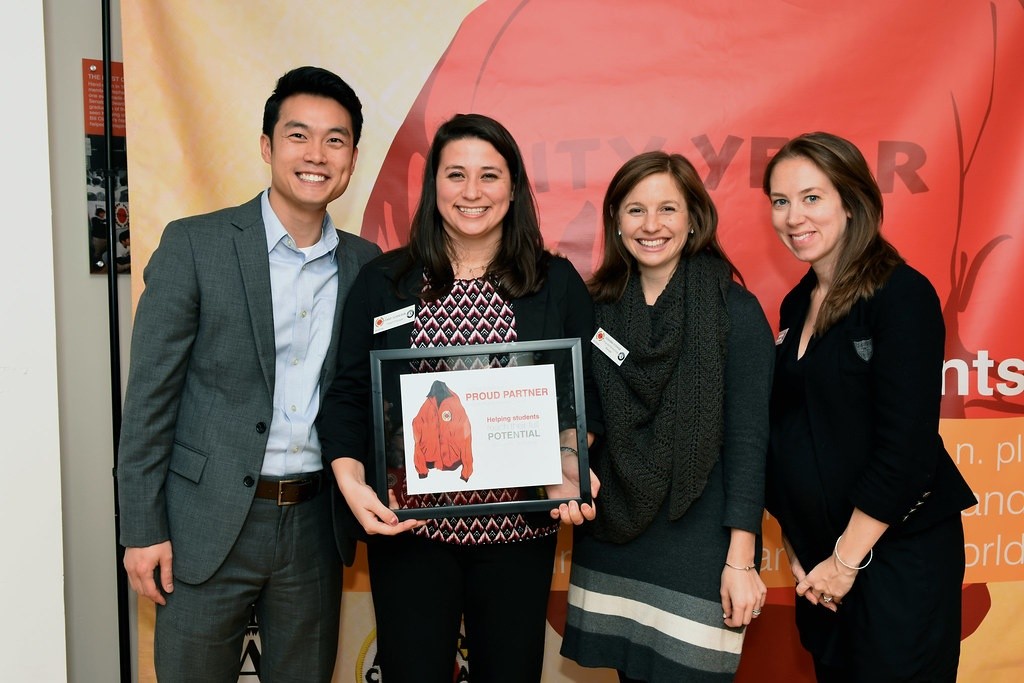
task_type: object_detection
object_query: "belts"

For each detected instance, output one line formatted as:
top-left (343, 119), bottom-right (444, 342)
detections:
top-left (255, 476), bottom-right (328, 504)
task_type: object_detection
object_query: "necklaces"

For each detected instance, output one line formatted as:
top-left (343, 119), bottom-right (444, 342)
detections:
top-left (450, 258), bottom-right (488, 278)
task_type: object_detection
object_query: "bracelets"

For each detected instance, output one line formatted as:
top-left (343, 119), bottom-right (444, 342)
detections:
top-left (560, 445), bottom-right (580, 457)
top-left (725, 562), bottom-right (756, 570)
top-left (834, 534), bottom-right (873, 569)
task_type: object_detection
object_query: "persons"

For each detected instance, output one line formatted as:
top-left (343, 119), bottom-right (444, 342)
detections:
top-left (761, 126), bottom-right (978, 683)
top-left (313, 112), bottom-right (594, 683)
top-left (113, 64), bottom-right (382, 683)
top-left (557, 151), bottom-right (774, 683)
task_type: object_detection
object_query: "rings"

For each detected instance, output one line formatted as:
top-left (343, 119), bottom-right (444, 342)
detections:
top-left (752, 610), bottom-right (761, 616)
top-left (822, 594), bottom-right (833, 603)
